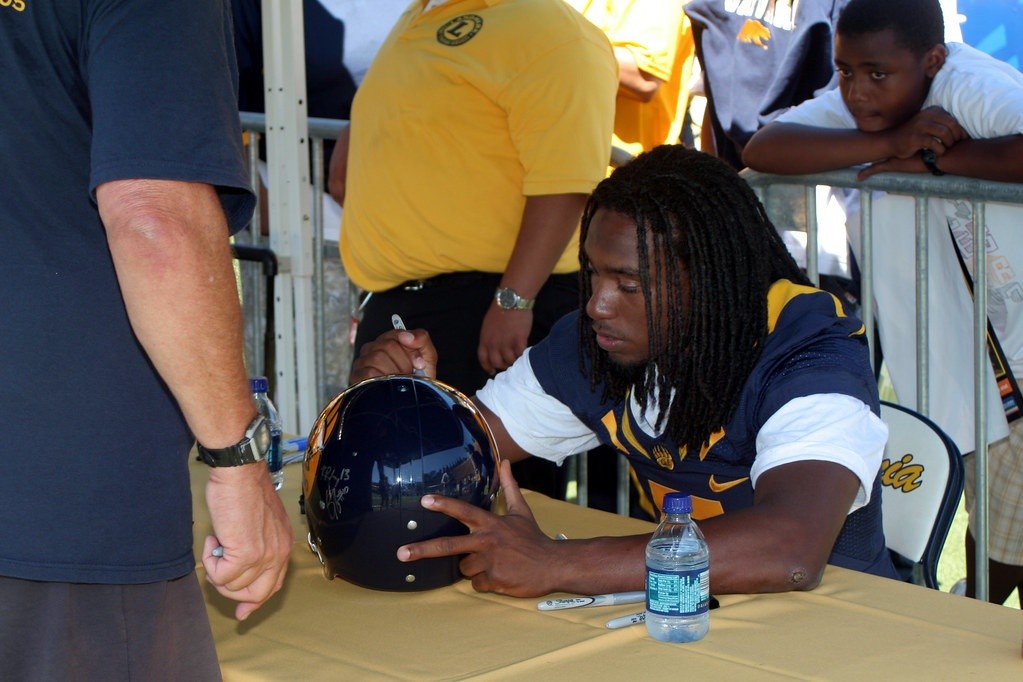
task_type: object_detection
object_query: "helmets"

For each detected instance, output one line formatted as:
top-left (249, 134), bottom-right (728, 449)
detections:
top-left (298, 375), bottom-right (502, 592)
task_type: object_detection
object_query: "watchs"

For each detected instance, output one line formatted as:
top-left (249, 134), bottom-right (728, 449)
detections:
top-left (919, 148), bottom-right (945, 177)
top-left (195, 413), bottom-right (274, 469)
top-left (493, 287), bottom-right (534, 313)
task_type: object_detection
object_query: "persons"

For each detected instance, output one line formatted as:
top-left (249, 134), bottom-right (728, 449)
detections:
top-left (0, 1), bottom-right (294, 682)
top-left (335, 0), bottom-right (623, 398)
top-left (202, 0), bottom-right (664, 244)
top-left (744, 2), bottom-right (1023, 612)
top-left (347, 143), bottom-right (899, 598)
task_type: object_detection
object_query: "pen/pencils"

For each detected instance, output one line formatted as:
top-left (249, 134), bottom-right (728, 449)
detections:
top-left (607, 611), bottom-right (646, 629)
top-left (392, 314), bottom-right (426, 375)
top-left (212, 546), bottom-right (223, 557)
top-left (538, 591), bottom-right (646, 610)
top-left (282, 452), bottom-right (304, 464)
top-left (282, 437), bottom-right (308, 451)
top-left (557, 532), bottom-right (567, 539)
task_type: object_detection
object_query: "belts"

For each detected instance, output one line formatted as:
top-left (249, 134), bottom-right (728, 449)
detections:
top-left (399, 278), bottom-right (430, 291)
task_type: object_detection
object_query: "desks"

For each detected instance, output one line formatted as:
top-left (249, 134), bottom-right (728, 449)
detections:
top-left (185, 433), bottom-right (1022, 680)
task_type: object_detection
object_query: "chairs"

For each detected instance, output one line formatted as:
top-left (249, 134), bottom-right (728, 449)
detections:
top-left (879, 402), bottom-right (964, 591)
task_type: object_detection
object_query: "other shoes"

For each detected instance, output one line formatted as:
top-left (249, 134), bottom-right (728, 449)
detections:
top-left (950, 577), bottom-right (966, 597)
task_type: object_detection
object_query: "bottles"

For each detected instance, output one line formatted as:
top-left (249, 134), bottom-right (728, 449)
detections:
top-left (248, 376), bottom-right (283, 490)
top-left (646, 492), bottom-right (710, 643)
